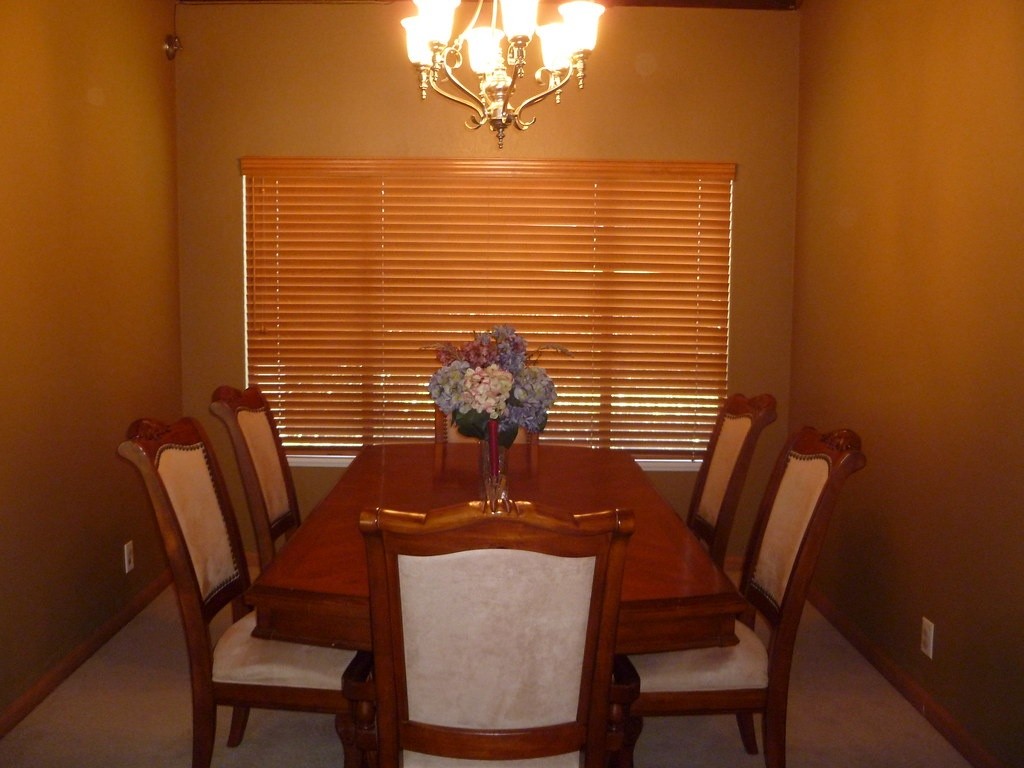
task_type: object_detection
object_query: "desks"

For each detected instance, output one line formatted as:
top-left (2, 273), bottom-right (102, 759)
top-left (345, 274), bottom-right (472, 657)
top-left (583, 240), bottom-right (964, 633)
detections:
top-left (251, 443), bottom-right (748, 652)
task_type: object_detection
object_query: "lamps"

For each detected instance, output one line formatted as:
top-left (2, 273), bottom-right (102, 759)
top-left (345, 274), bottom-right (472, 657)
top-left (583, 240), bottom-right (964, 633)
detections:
top-left (399, 0), bottom-right (608, 142)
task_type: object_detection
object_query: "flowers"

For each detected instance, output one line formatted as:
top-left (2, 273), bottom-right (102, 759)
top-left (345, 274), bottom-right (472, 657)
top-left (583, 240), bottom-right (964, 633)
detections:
top-left (423, 325), bottom-right (559, 448)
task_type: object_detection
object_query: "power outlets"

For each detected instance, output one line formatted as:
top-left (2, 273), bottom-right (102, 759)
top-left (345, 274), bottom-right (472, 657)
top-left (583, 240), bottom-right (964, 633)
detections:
top-left (124, 540), bottom-right (134, 574)
top-left (920, 616), bottom-right (935, 660)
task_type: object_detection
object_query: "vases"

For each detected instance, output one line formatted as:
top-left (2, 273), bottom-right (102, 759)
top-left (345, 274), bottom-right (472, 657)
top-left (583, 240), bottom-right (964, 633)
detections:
top-left (479, 440), bottom-right (513, 516)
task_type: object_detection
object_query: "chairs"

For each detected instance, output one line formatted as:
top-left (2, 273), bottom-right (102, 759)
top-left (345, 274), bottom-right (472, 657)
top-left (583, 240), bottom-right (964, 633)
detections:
top-left (685, 390), bottom-right (778, 569)
top-left (334, 499), bottom-right (644, 768)
top-left (116, 415), bottom-right (378, 768)
top-left (628, 425), bottom-right (866, 768)
top-left (208, 384), bottom-right (302, 570)
top-left (434, 401), bottom-right (541, 445)
